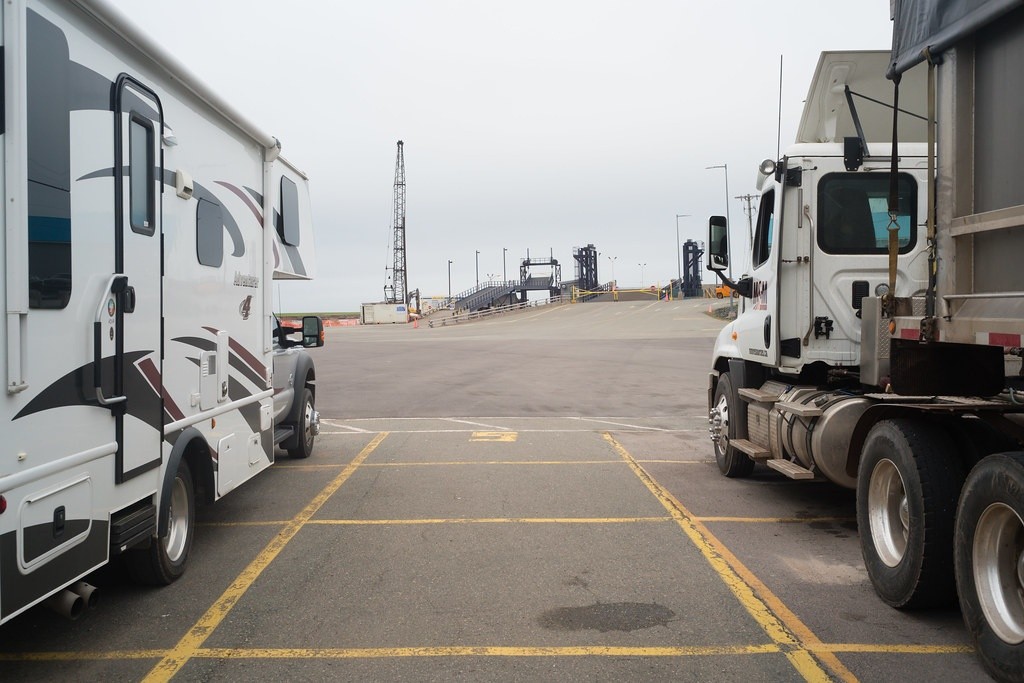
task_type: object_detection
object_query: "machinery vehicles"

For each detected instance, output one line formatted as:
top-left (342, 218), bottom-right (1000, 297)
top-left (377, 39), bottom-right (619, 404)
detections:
top-left (381, 140), bottom-right (421, 316)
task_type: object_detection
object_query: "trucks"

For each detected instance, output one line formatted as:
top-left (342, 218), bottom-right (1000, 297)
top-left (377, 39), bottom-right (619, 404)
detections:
top-left (704, 2), bottom-right (1024, 683)
top-left (0, 0), bottom-right (328, 632)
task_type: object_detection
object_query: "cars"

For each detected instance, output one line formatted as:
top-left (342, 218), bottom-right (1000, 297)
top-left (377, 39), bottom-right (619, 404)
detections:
top-left (714, 277), bottom-right (739, 299)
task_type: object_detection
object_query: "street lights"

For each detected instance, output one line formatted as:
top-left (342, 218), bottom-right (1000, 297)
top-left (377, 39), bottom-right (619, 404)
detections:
top-left (638, 263), bottom-right (647, 290)
top-left (447, 260), bottom-right (452, 304)
top-left (502, 247), bottom-right (509, 285)
top-left (608, 256), bottom-right (618, 286)
top-left (676, 213), bottom-right (693, 292)
top-left (475, 250), bottom-right (481, 291)
